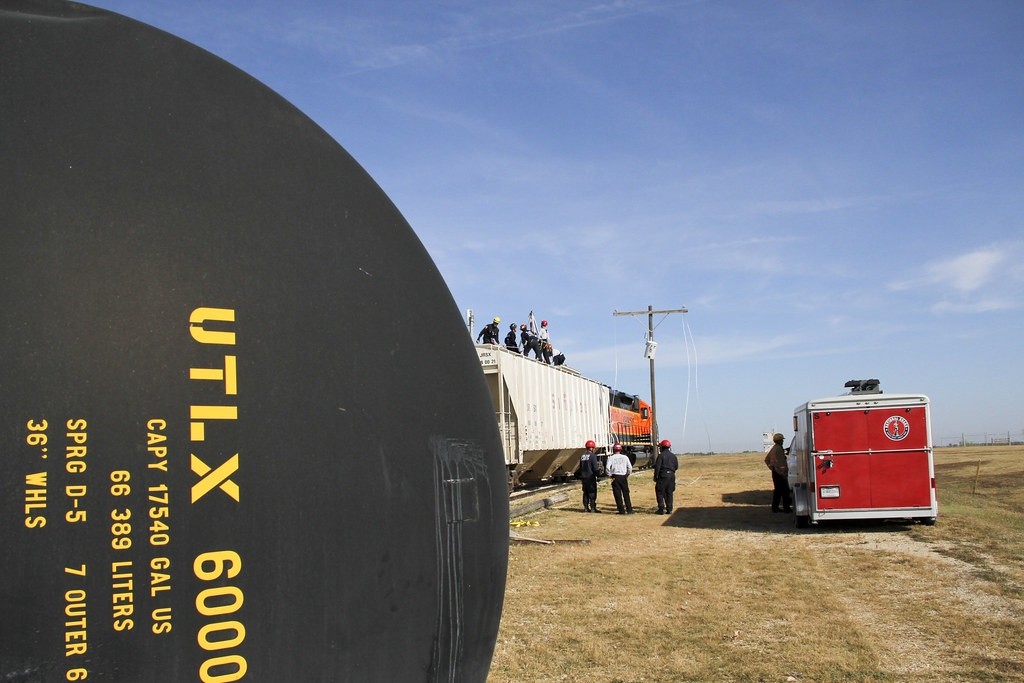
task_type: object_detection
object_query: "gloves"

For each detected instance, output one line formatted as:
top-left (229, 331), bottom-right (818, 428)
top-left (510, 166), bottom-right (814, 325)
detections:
top-left (476, 340), bottom-right (480, 344)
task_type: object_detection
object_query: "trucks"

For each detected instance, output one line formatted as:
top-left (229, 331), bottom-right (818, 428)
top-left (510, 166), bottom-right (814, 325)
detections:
top-left (784, 378), bottom-right (938, 530)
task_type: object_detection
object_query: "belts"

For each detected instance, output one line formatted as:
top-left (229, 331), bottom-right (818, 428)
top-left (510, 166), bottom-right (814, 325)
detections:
top-left (665, 471), bottom-right (673, 473)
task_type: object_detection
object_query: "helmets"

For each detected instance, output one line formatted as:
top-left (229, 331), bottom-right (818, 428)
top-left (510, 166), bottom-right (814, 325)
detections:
top-left (520, 324), bottom-right (526, 330)
top-left (542, 321), bottom-right (547, 325)
top-left (613, 444), bottom-right (622, 450)
top-left (773, 434), bottom-right (786, 441)
top-left (493, 317), bottom-right (500, 323)
top-left (661, 440), bottom-right (670, 447)
top-left (510, 323), bottom-right (516, 329)
top-left (585, 441), bottom-right (595, 448)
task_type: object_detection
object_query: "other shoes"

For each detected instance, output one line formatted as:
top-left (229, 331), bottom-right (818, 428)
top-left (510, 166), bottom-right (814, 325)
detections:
top-left (784, 508), bottom-right (793, 513)
top-left (585, 508), bottom-right (591, 512)
top-left (655, 510), bottom-right (663, 513)
top-left (666, 511), bottom-right (672, 514)
top-left (592, 508), bottom-right (601, 512)
top-left (772, 507), bottom-right (784, 513)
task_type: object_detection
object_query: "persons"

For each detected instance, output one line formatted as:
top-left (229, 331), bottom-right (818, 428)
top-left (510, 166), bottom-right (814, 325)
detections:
top-left (653, 440), bottom-right (679, 515)
top-left (579, 440), bottom-right (601, 513)
top-left (505, 323), bottom-right (520, 353)
top-left (551, 348), bottom-right (566, 366)
top-left (520, 324), bottom-right (543, 361)
top-left (764, 433), bottom-right (793, 513)
top-left (537, 320), bottom-right (551, 365)
top-left (476, 317), bottom-right (500, 345)
top-left (606, 443), bottom-right (633, 515)
